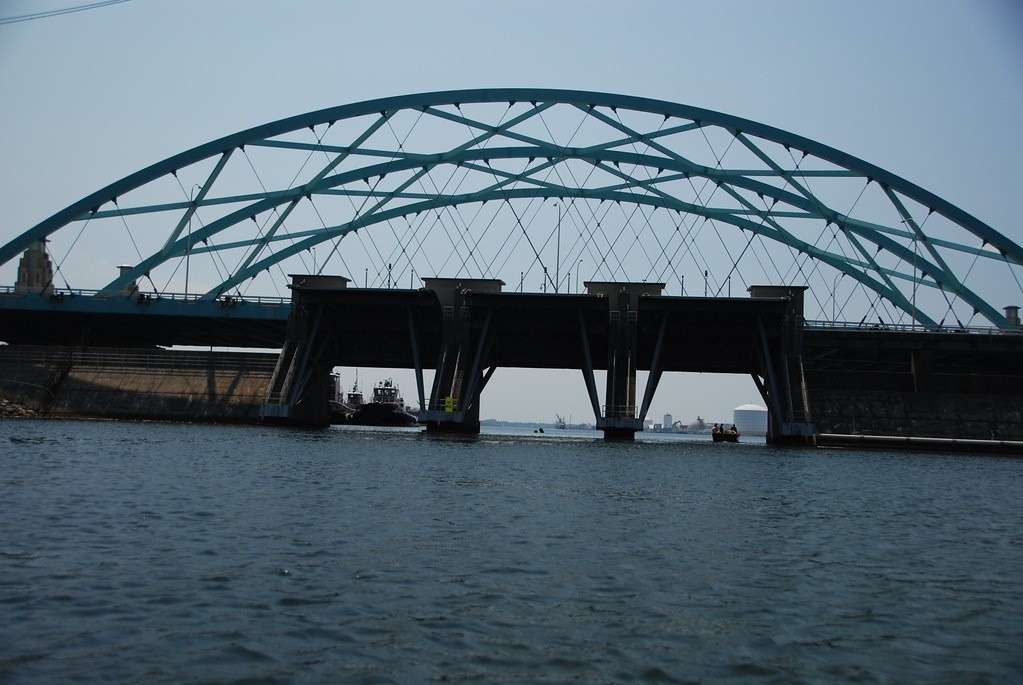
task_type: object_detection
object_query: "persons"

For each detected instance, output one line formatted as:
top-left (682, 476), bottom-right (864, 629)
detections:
top-left (719, 424), bottom-right (723, 434)
top-left (711, 422), bottom-right (720, 434)
top-left (730, 424), bottom-right (737, 434)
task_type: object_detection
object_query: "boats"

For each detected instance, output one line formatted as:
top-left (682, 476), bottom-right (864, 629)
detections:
top-left (711, 431), bottom-right (741, 443)
top-left (330, 367), bottom-right (427, 427)
